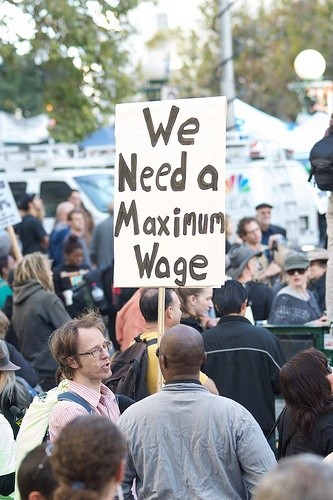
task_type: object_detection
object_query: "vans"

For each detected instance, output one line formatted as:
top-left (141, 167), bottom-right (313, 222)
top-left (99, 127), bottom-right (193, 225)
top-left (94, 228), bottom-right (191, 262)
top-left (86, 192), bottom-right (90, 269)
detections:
top-left (0, 168), bottom-right (118, 252)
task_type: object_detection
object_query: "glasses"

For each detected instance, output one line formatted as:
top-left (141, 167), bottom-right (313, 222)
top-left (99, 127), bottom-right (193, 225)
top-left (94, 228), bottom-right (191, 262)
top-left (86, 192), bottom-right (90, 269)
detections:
top-left (325, 364), bottom-right (332, 376)
top-left (287, 268), bottom-right (307, 276)
top-left (77, 340), bottom-right (113, 357)
top-left (156, 347), bottom-right (166, 357)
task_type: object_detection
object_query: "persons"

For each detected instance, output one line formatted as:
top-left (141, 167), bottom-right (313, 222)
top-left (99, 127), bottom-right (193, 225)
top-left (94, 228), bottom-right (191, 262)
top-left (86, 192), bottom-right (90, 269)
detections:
top-left (316, 112), bottom-right (333, 249)
top-left (249, 202), bottom-right (289, 247)
top-left (46, 308), bottom-right (128, 500)
top-left (267, 251), bottom-right (328, 330)
top-left (273, 348), bottom-right (333, 462)
top-left (174, 284), bottom-right (219, 336)
top-left (0, 186), bottom-right (333, 500)
top-left (15, 442), bottom-right (60, 500)
top-left (305, 242), bottom-right (329, 317)
top-left (109, 322), bottom-right (284, 499)
top-left (61, 210), bottom-right (95, 270)
top-left (9, 250), bottom-right (76, 392)
top-left (8, 191), bottom-right (52, 260)
top-left (108, 286), bottom-right (222, 399)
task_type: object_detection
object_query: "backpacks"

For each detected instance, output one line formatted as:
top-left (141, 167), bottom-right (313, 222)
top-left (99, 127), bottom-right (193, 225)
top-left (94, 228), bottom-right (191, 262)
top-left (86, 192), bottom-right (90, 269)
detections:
top-left (14, 386), bottom-right (95, 500)
top-left (102, 333), bottom-right (157, 414)
top-left (309, 125), bottom-right (333, 191)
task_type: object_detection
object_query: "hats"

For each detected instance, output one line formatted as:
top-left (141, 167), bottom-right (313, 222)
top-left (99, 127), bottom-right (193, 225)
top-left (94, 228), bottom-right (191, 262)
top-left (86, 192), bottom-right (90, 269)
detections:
top-left (0, 340), bottom-right (21, 371)
top-left (256, 203), bottom-right (272, 209)
top-left (17, 193), bottom-right (36, 211)
top-left (284, 253), bottom-right (310, 271)
top-left (307, 247), bottom-right (329, 261)
top-left (225, 243), bottom-right (263, 277)
top-left (0, 234), bottom-right (19, 257)
